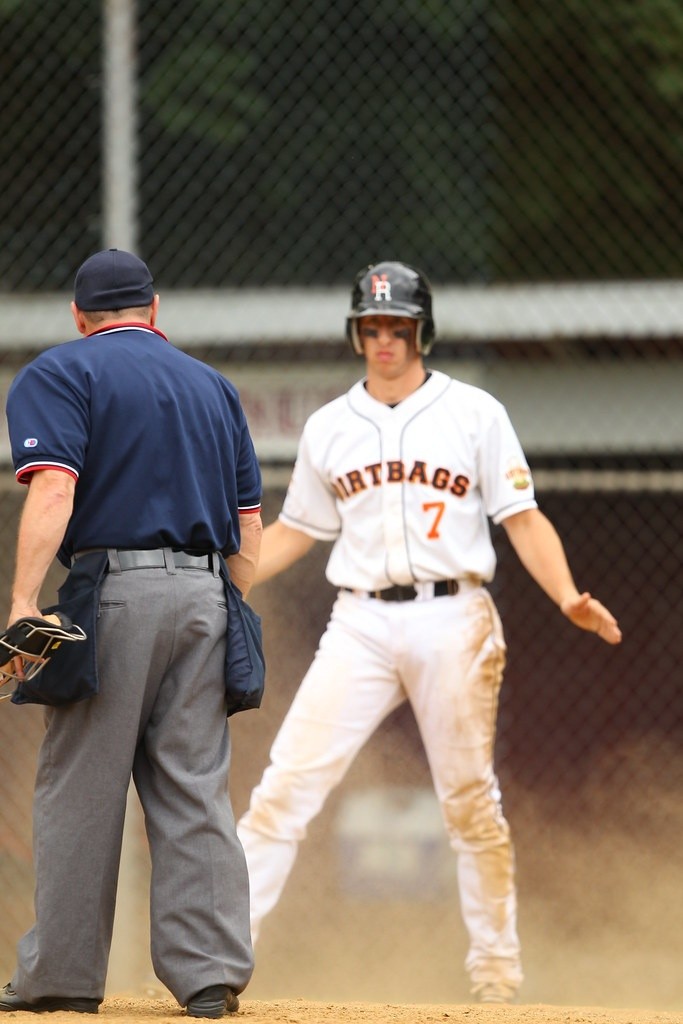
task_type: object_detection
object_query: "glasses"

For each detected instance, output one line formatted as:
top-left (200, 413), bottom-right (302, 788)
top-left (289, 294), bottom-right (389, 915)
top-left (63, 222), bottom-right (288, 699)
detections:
top-left (357, 326), bottom-right (413, 340)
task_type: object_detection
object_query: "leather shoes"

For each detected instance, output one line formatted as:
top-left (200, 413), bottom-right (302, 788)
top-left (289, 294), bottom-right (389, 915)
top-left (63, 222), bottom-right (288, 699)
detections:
top-left (0, 989), bottom-right (98, 1015)
top-left (109, 549), bottom-right (214, 573)
top-left (187, 986), bottom-right (240, 1018)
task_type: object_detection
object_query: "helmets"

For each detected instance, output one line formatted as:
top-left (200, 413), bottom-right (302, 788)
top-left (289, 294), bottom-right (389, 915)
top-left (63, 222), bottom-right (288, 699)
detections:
top-left (347, 261), bottom-right (434, 356)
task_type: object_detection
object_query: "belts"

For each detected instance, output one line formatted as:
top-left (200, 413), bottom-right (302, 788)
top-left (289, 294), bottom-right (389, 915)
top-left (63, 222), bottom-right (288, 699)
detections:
top-left (345, 579), bottom-right (458, 601)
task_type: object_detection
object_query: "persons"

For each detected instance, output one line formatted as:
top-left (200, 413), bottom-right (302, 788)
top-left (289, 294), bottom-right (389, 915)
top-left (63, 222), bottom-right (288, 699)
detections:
top-left (237, 259), bottom-right (622, 1004)
top-left (0, 247), bottom-right (264, 1017)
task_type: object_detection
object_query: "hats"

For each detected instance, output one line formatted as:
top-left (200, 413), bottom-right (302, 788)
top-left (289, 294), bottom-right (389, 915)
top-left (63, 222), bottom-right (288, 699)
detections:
top-left (75, 248), bottom-right (154, 311)
